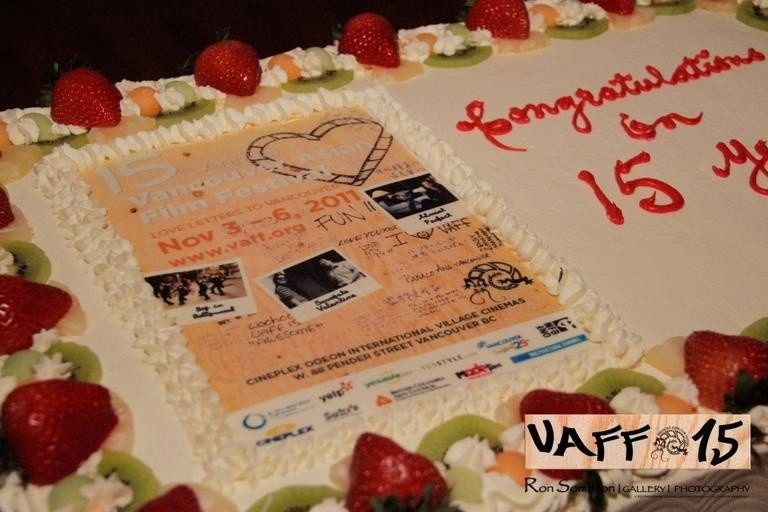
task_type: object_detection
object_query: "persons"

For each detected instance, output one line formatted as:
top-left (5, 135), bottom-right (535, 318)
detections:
top-left (273, 271), bottom-right (308, 308)
top-left (420, 180), bottom-right (456, 205)
top-left (154, 265), bottom-right (229, 305)
top-left (320, 258), bottom-right (363, 287)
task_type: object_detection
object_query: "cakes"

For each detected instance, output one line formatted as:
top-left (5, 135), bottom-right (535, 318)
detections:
top-left (0, 0), bottom-right (768, 512)
